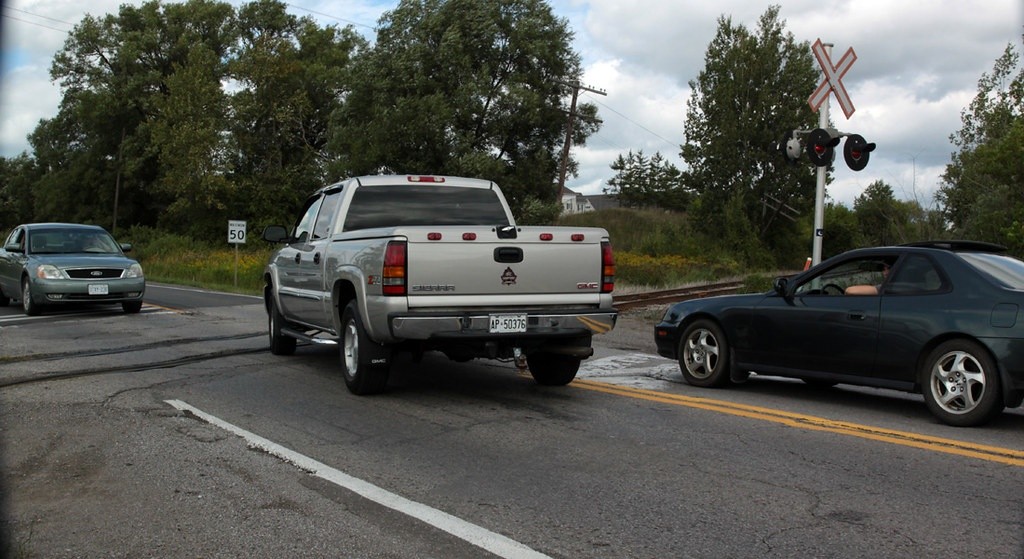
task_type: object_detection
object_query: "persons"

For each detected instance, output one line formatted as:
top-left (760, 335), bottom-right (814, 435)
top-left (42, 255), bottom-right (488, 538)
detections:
top-left (845, 255), bottom-right (899, 295)
top-left (76, 232), bottom-right (98, 252)
top-left (33, 234), bottom-right (46, 249)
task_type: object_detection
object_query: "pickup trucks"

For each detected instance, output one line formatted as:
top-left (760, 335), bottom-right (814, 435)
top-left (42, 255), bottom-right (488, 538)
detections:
top-left (261, 174), bottom-right (619, 396)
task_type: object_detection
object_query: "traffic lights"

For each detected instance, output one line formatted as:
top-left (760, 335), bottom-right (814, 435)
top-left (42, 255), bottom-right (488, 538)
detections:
top-left (843, 134), bottom-right (877, 172)
top-left (807, 128), bottom-right (841, 167)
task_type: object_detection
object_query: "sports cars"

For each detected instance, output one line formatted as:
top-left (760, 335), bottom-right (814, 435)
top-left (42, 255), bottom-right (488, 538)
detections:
top-left (654, 241), bottom-right (1023, 426)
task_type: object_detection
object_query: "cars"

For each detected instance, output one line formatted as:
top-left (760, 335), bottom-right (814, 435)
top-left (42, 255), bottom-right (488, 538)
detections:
top-left (0, 223), bottom-right (147, 315)
